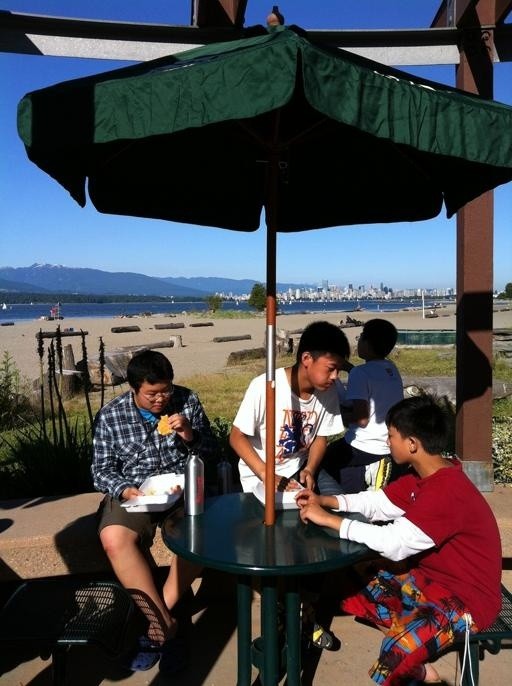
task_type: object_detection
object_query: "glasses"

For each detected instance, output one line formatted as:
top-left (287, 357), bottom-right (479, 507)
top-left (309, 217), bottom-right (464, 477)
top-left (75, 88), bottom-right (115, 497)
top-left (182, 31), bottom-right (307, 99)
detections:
top-left (139, 387), bottom-right (174, 404)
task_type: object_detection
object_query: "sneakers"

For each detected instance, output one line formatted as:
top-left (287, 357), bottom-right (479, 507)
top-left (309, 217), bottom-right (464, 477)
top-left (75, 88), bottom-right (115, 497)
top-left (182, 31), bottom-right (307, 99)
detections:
top-left (431, 651), bottom-right (462, 686)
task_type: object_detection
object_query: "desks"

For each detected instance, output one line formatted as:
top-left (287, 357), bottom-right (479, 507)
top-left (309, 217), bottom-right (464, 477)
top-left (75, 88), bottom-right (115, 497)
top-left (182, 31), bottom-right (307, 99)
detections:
top-left (160, 488), bottom-right (368, 686)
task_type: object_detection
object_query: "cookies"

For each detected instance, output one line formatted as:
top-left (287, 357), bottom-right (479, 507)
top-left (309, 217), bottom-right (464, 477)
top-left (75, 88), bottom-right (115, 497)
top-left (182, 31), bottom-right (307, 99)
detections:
top-left (157, 415), bottom-right (174, 437)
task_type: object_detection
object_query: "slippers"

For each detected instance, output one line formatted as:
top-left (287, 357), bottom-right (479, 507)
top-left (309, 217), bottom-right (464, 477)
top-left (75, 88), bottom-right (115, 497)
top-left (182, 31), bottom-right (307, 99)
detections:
top-left (161, 639), bottom-right (188, 676)
top-left (303, 621), bottom-right (335, 650)
top-left (128, 634), bottom-right (160, 672)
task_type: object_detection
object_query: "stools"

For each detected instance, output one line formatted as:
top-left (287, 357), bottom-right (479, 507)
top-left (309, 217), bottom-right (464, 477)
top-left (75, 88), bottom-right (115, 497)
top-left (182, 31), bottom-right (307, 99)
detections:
top-left (0, 576), bottom-right (136, 686)
top-left (449, 576), bottom-right (512, 686)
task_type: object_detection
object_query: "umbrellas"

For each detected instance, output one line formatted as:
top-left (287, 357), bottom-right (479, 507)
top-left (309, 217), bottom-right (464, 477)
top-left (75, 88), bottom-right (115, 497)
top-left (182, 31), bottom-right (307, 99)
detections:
top-left (17, 4), bottom-right (511, 524)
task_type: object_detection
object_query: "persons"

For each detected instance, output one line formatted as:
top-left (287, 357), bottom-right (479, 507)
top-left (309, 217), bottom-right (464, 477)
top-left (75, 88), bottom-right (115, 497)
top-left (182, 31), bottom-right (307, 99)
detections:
top-left (294, 394), bottom-right (504, 685)
top-left (228, 321), bottom-right (349, 651)
top-left (88, 348), bottom-right (222, 673)
top-left (317, 318), bottom-right (404, 496)
